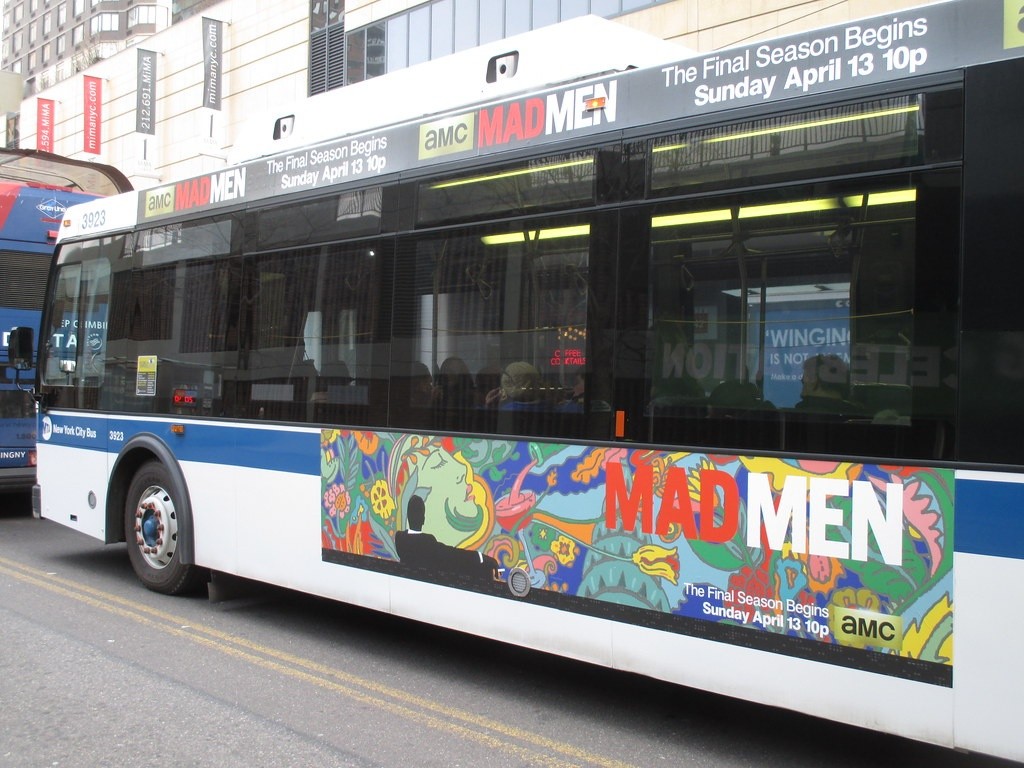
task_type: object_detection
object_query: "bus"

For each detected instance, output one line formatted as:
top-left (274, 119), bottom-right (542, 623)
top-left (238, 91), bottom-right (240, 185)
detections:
top-left (7, 0), bottom-right (1022, 768)
top-left (0, 183), bottom-right (105, 508)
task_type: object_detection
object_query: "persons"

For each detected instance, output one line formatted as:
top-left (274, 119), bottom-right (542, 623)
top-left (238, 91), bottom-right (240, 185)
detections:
top-left (651, 376), bottom-right (687, 409)
top-left (707, 382), bottom-right (758, 417)
top-left (412, 358), bottom-right (540, 410)
top-left (559, 367), bottom-right (584, 407)
top-left (796, 357), bottom-right (864, 413)
top-left (291, 360), bottom-right (371, 402)
